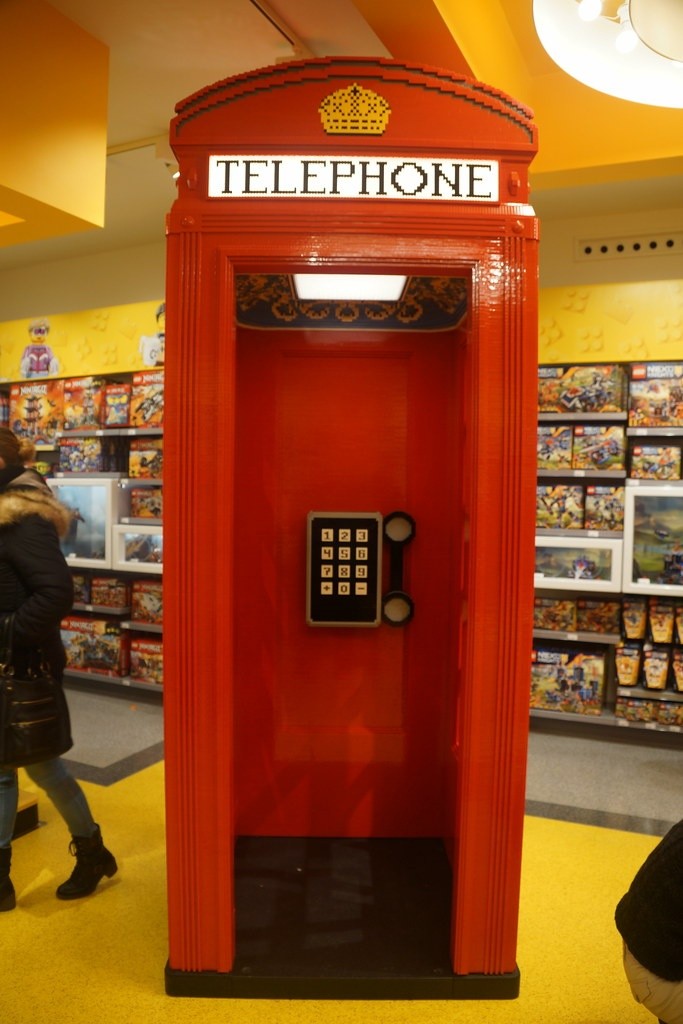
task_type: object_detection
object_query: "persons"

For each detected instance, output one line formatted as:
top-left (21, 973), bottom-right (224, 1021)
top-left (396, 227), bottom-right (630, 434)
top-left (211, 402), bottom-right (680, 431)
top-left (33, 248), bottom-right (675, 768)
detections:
top-left (614, 819), bottom-right (683, 1024)
top-left (0, 423), bottom-right (119, 911)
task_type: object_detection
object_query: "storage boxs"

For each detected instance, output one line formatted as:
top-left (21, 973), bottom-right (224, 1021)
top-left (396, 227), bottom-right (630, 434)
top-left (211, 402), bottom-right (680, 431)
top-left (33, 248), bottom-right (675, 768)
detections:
top-left (527, 364), bottom-right (683, 731)
top-left (0, 370), bottom-right (165, 689)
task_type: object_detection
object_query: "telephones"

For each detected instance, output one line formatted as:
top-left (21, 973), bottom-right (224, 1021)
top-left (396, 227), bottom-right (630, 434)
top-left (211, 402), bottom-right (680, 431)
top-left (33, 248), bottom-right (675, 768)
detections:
top-left (304, 510), bottom-right (416, 628)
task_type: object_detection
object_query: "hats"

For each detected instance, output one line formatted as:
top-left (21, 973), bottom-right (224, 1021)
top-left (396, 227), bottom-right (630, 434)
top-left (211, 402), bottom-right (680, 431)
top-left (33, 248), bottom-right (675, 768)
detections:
top-left (0, 426), bottom-right (36, 468)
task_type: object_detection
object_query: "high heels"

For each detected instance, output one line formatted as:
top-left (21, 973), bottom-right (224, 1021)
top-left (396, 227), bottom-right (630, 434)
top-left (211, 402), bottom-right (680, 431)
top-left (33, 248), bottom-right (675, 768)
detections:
top-left (56, 824), bottom-right (118, 900)
top-left (0, 847), bottom-right (16, 911)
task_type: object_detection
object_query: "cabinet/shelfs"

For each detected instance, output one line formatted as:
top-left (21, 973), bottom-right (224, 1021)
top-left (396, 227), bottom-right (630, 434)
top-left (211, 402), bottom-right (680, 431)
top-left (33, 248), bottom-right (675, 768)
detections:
top-left (527, 360), bottom-right (683, 739)
top-left (0, 368), bottom-right (167, 695)
